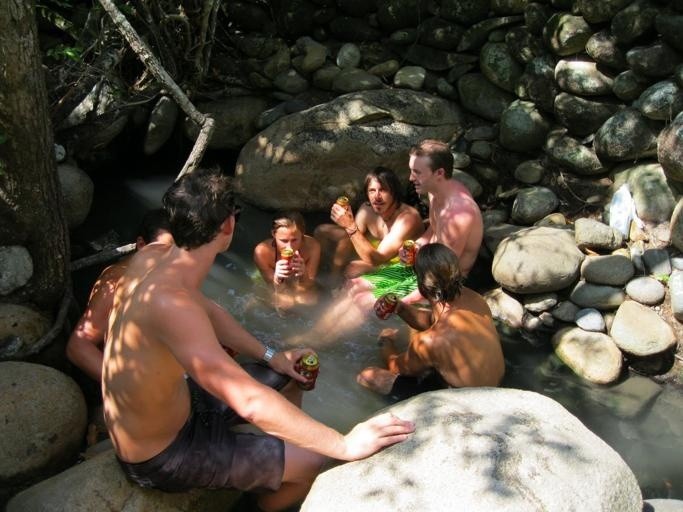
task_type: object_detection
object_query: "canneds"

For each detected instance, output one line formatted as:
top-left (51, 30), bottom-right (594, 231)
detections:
top-left (403, 239), bottom-right (416, 267)
top-left (336, 196), bottom-right (349, 212)
top-left (296, 353), bottom-right (320, 391)
top-left (281, 249), bottom-right (293, 276)
top-left (375, 293), bottom-right (397, 320)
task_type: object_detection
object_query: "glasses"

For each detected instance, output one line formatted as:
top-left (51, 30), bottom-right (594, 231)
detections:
top-left (232, 205), bottom-right (241, 223)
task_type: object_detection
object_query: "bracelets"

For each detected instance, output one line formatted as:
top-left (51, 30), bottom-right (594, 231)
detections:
top-left (257, 345), bottom-right (276, 367)
top-left (344, 226), bottom-right (360, 235)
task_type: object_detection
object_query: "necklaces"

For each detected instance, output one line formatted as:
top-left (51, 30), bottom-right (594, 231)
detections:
top-left (378, 212), bottom-right (395, 228)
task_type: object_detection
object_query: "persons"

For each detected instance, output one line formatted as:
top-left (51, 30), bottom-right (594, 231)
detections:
top-left (64, 211), bottom-right (319, 427)
top-left (357, 242), bottom-right (506, 401)
top-left (282, 136), bottom-right (485, 349)
top-left (246, 209), bottom-right (323, 317)
top-left (100, 165), bottom-right (416, 512)
top-left (310, 167), bottom-right (427, 277)
